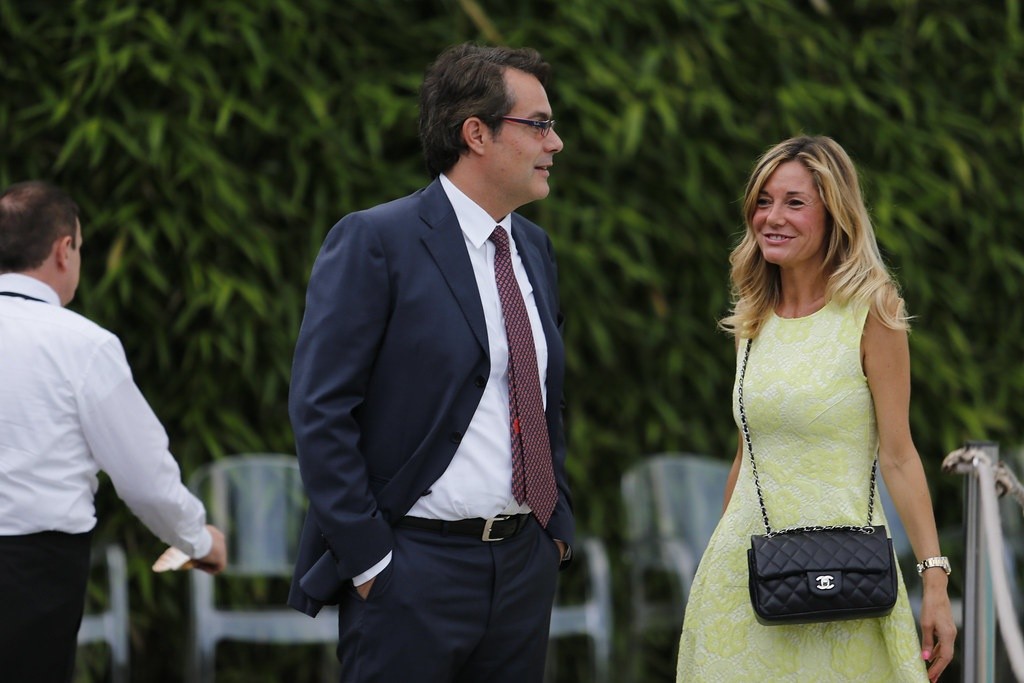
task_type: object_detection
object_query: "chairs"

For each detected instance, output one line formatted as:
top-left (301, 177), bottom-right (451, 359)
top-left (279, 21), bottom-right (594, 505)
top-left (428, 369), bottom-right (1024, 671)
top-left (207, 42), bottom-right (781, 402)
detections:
top-left (547, 453), bottom-right (735, 683)
top-left (77, 543), bottom-right (132, 683)
top-left (184, 453), bottom-right (342, 683)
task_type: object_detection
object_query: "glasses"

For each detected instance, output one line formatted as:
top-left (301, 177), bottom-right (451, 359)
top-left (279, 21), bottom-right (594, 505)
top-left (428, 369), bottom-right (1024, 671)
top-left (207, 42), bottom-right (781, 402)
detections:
top-left (493, 114), bottom-right (556, 138)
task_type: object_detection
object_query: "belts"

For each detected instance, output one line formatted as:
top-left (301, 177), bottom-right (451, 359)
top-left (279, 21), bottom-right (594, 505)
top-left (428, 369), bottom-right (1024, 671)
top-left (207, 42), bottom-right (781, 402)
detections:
top-left (400, 512), bottom-right (530, 542)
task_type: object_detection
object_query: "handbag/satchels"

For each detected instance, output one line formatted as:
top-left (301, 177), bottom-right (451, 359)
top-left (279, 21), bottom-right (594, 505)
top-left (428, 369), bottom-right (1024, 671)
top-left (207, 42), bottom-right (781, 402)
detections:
top-left (746, 524), bottom-right (900, 626)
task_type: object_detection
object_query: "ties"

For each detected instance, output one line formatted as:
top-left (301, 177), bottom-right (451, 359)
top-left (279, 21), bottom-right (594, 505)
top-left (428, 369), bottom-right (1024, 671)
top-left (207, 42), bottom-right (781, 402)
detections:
top-left (490, 227), bottom-right (559, 529)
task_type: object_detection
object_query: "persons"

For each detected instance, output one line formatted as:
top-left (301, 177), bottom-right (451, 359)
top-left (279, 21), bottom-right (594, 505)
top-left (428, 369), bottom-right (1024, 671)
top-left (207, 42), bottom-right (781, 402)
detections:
top-left (675, 135), bottom-right (957, 683)
top-left (291, 44), bottom-right (575, 683)
top-left (0, 182), bottom-right (228, 683)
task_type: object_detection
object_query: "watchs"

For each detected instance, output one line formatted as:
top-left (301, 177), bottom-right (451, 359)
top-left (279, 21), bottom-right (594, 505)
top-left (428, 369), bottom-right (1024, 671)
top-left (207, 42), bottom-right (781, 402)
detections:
top-left (917, 556), bottom-right (952, 576)
